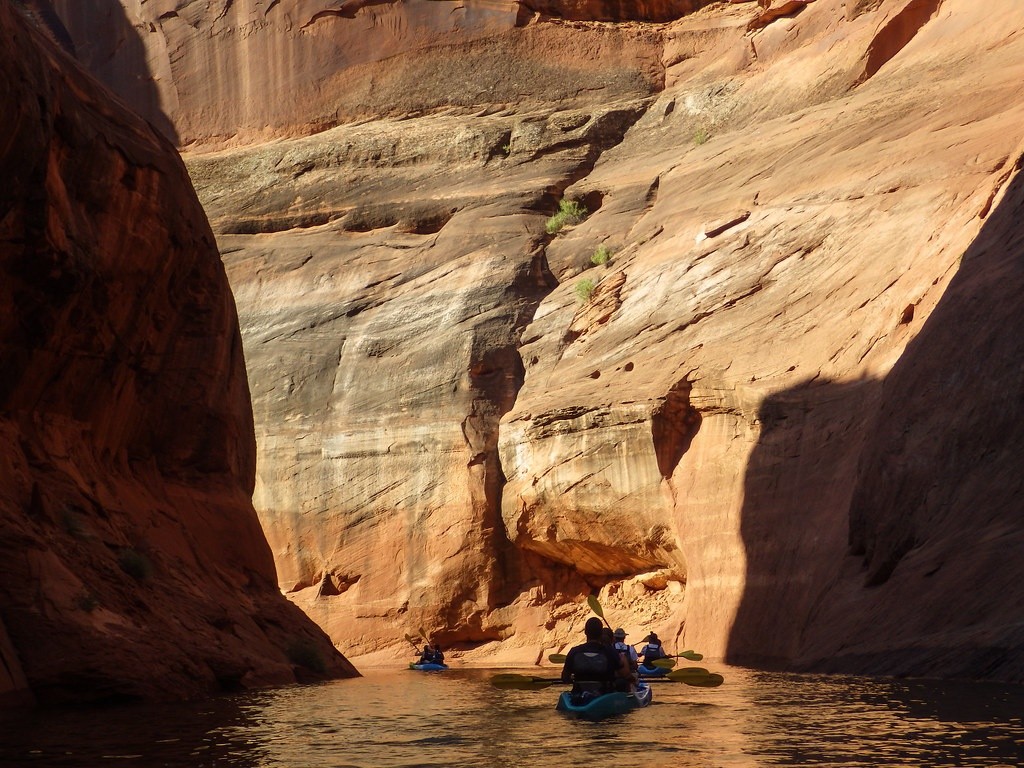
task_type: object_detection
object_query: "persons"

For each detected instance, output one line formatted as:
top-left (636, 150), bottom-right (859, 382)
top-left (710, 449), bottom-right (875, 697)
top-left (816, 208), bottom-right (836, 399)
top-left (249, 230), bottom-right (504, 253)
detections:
top-left (561, 617), bottom-right (629, 697)
top-left (414, 642), bottom-right (444, 665)
top-left (631, 633), bottom-right (673, 669)
top-left (612, 628), bottom-right (639, 672)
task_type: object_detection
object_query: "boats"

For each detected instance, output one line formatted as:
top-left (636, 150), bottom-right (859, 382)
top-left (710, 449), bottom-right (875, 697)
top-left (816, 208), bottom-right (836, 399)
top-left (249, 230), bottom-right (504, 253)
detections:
top-left (410, 660), bottom-right (448, 671)
top-left (556, 679), bottom-right (652, 718)
top-left (635, 662), bottom-right (672, 678)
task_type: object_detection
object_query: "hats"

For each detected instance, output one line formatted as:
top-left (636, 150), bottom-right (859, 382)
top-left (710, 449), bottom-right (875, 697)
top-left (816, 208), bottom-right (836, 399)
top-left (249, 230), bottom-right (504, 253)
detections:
top-left (613, 627), bottom-right (628, 637)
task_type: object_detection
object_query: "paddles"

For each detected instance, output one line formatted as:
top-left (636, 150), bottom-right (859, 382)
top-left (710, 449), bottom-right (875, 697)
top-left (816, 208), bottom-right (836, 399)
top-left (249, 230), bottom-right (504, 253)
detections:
top-left (491, 595), bottom-right (725, 692)
top-left (418, 626), bottom-right (434, 650)
top-left (404, 632), bottom-right (422, 656)
